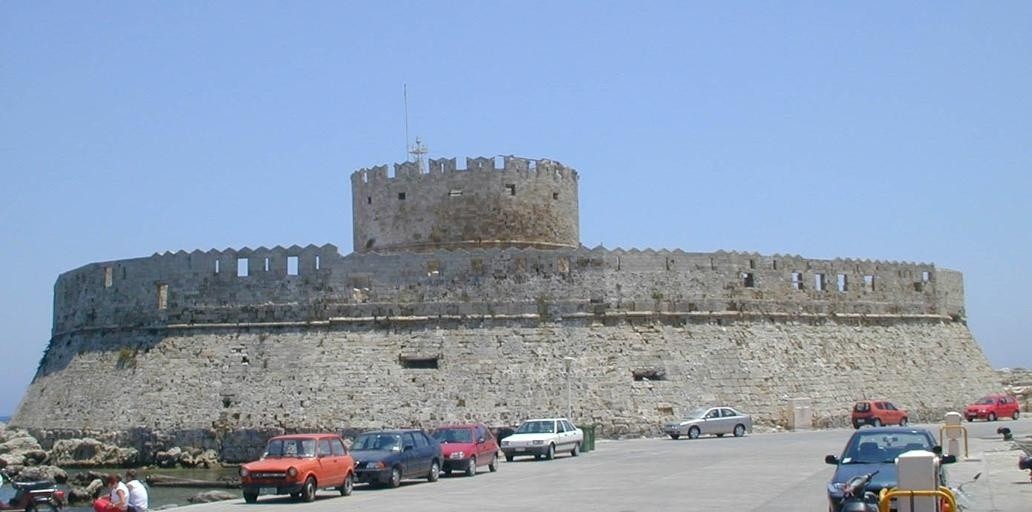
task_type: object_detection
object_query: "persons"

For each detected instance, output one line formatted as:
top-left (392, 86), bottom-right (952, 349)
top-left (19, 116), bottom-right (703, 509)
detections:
top-left (69, 469), bottom-right (149, 512)
top-left (933, 446), bottom-right (942, 455)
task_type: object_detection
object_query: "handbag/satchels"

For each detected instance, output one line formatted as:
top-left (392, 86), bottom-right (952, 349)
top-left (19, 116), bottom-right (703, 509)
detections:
top-left (95, 498), bottom-right (121, 512)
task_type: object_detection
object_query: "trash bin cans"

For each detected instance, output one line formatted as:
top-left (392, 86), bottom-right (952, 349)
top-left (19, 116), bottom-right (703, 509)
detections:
top-left (576, 424), bottom-right (597, 453)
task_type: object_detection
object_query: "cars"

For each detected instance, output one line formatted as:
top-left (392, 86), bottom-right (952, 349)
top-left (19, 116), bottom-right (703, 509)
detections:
top-left (826, 426), bottom-right (956, 512)
top-left (664, 406), bottom-right (752, 439)
top-left (964, 393), bottom-right (1020, 421)
top-left (851, 400), bottom-right (908, 429)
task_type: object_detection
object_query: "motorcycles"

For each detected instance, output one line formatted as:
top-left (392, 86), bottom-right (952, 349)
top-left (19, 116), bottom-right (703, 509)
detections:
top-left (833, 470), bottom-right (879, 512)
top-left (0, 469), bottom-right (65, 512)
